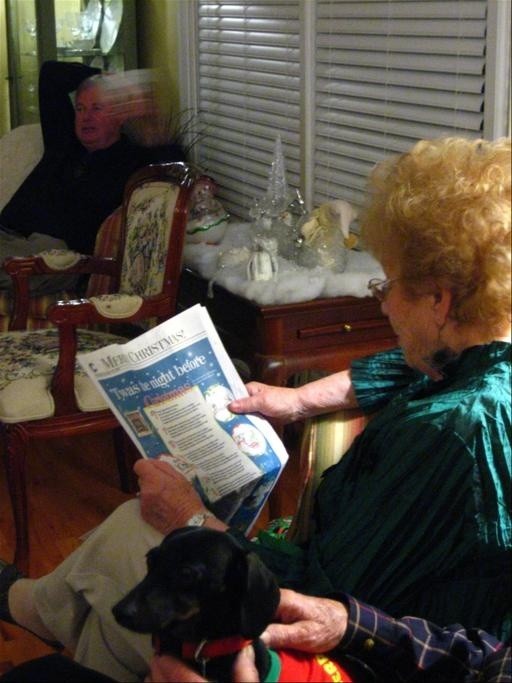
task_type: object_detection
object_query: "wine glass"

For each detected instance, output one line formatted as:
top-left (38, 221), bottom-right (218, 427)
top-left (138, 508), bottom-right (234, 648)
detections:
top-left (23, 10), bottom-right (80, 55)
top-left (22, 69), bottom-right (39, 111)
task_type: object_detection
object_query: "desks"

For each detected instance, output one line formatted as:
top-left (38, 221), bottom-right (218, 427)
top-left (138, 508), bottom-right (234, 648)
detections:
top-left (177, 221), bottom-right (399, 520)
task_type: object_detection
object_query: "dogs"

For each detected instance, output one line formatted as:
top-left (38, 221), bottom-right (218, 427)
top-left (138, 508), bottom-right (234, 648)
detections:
top-left (110, 525), bottom-right (380, 683)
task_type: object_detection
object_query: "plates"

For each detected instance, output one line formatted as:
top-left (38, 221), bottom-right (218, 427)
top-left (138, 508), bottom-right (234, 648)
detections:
top-left (81, 0), bottom-right (102, 40)
top-left (98, 0), bottom-right (124, 53)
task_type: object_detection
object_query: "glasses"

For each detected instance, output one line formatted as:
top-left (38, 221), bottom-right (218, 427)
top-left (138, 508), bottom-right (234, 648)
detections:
top-left (369, 277), bottom-right (400, 300)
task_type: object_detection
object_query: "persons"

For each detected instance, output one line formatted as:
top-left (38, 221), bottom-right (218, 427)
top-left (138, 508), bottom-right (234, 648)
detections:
top-left (0, 57), bottom-right (190, 292)
top-left (0, 133), bottom-right (511, 682)
top-left (147, 588), bottom-right (511, 681)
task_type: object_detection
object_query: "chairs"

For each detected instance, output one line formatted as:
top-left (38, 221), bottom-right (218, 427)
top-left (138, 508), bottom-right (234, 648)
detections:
top-left (1, 160), bottom-right (192, 571)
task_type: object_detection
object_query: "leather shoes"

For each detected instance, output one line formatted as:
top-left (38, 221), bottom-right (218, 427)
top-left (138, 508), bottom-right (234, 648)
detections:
top-left (0, 562), bottom-right (65, 654)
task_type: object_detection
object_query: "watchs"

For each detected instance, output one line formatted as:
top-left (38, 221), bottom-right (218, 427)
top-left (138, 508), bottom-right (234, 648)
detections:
top-left (186, 511), bottom-right (215, 527)
